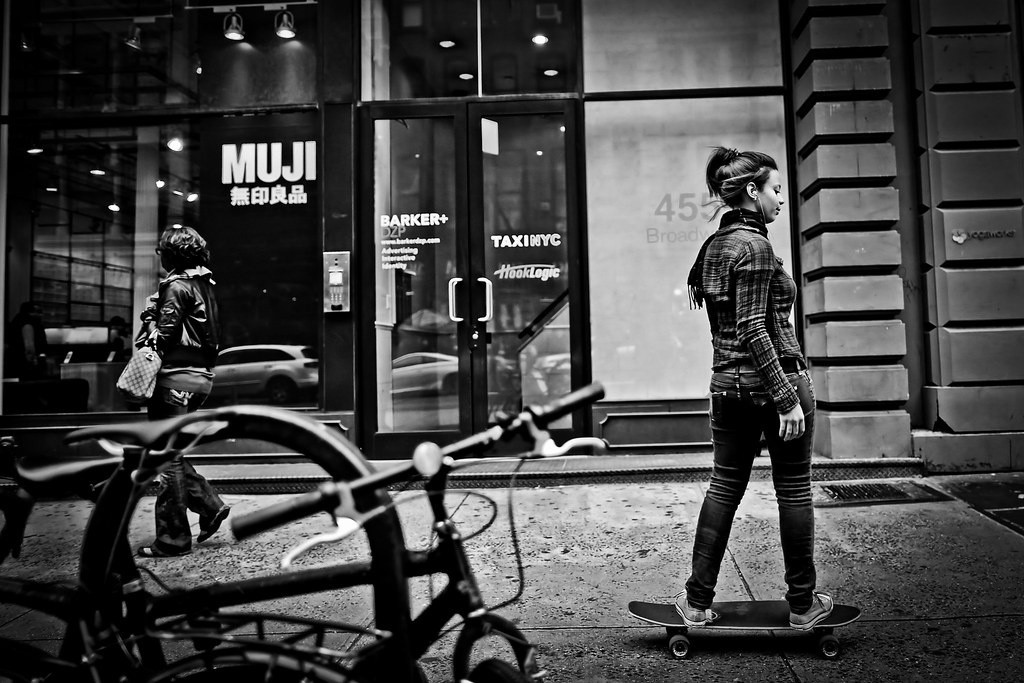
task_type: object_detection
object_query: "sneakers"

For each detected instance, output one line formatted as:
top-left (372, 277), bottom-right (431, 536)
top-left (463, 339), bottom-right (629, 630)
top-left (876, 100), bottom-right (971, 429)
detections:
top-left (674, 590), bottom-right (708, 627)
top-left (789, 594), bottom-right (833, 630)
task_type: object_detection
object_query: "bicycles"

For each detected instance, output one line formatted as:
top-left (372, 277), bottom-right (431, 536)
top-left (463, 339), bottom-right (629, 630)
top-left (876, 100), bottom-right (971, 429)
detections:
top-left (0, 376), bottom-right (615, 683)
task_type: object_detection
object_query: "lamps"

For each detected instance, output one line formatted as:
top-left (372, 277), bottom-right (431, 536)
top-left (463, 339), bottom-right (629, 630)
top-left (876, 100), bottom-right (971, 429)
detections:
top-left (125, 23), bottom-right (143, 49)
top-left (274, 11), bottom-right (296, 38)
top-left (223, 8), bottom-right (245, 40)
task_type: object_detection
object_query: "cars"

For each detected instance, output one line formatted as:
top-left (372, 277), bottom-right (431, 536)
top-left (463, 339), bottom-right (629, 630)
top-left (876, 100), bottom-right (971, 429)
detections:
top-left (210, 344), bottom-right (321, 404)
top-left (389, 351), bottom-right (463, 397)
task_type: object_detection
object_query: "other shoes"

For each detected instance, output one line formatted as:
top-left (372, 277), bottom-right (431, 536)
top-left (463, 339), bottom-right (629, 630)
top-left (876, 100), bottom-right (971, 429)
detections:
top-left (139, 541), bottom-right (193, 558)
top-left (197, 502), bottom-right (231, 541)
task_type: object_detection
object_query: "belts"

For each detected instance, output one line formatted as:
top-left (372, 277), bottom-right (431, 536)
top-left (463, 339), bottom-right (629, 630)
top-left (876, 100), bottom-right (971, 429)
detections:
top-left (718, 357), bottom-right (806, 373)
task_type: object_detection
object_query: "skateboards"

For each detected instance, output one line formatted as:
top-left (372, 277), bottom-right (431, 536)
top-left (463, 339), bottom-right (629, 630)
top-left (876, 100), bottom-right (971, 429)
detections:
top-left (626, 599), bottom-right (864, 658)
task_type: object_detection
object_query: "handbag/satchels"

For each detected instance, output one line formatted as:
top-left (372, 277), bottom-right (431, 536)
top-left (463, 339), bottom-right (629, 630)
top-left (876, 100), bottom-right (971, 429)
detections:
top-left (115, 329), bottom-right (160, 403)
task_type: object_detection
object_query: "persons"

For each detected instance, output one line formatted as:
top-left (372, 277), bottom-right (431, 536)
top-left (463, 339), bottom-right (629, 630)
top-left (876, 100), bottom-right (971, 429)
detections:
top-left (674, 147), bottom-right (834, 632)
top-left (139, 226), bottom-right (230, 557)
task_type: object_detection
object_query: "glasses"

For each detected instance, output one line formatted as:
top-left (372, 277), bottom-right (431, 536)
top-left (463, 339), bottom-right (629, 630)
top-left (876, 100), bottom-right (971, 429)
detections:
top-left (155, 246), bottom-right (162, 255)
